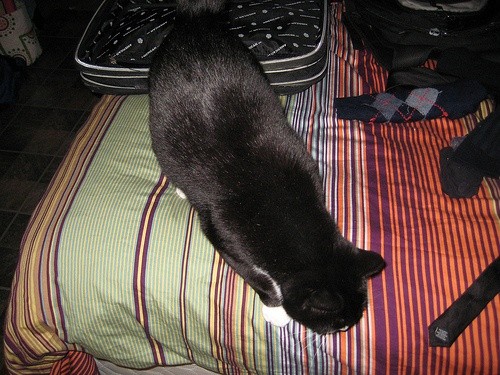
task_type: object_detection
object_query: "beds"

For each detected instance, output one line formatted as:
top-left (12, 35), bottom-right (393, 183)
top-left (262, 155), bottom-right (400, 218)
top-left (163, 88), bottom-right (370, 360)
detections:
top-left (4, 1), bottom-right (499, 374)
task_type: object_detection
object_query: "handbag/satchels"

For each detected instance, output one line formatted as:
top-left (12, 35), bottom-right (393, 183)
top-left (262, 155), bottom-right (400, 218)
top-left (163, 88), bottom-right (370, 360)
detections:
top-left (74, 0), bottom-right (332, 96)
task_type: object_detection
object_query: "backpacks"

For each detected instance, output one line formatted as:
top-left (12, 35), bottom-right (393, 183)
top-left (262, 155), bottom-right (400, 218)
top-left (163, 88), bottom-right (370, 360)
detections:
top-left (341, 0), bottom-right (500, 72)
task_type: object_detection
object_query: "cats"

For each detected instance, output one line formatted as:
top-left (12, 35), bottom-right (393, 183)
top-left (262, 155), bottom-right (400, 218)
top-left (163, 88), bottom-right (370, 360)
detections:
top-left (147, 0), bottom-right (388, 363)
